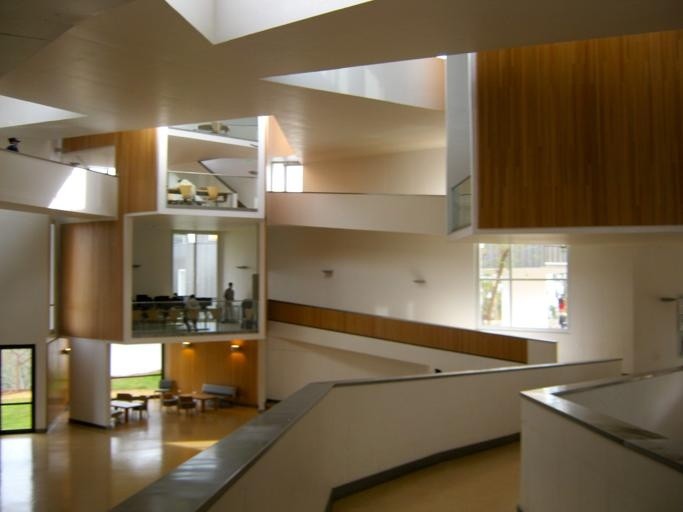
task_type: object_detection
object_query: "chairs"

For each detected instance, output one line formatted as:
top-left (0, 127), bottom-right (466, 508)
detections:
top-left (110, 120), bottom-right (257, 425)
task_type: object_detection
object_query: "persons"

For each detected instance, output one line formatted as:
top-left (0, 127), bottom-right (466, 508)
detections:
top-left (181, 293), bottom-right (200, 333)
top-left (221, 281), bottom-right (236, 323)
